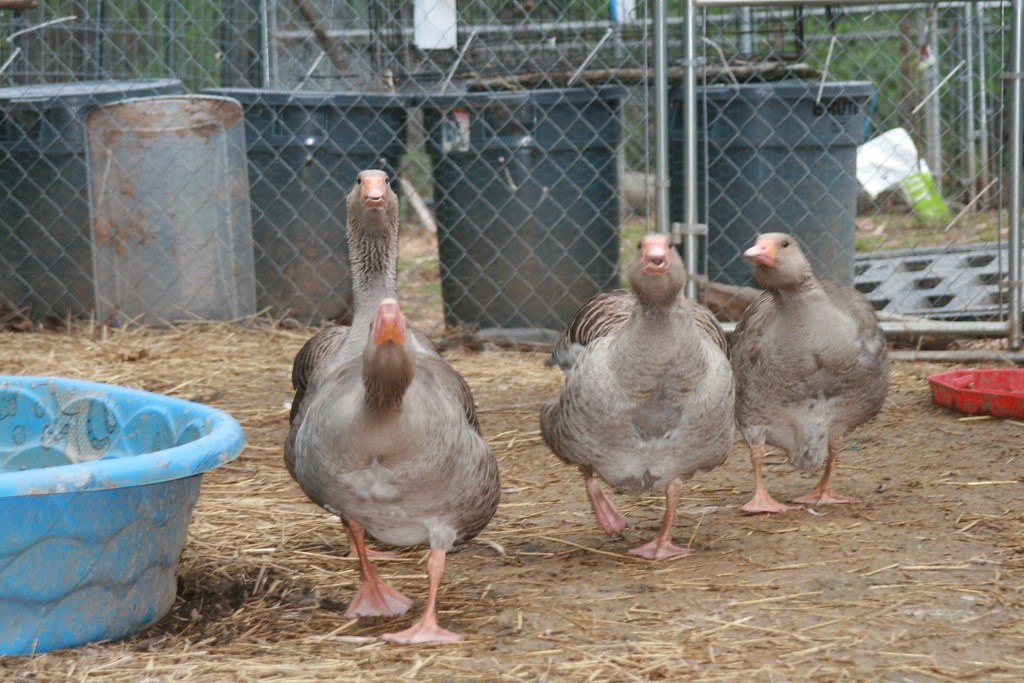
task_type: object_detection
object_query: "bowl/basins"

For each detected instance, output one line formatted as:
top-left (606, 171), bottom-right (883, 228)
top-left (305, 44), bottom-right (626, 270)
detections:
top-left (0, 376), bottom-right (244, 656)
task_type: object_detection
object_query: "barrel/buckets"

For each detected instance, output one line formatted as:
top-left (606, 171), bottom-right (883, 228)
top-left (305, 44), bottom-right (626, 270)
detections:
top-left (0, 76), bottom-right (182, 329)
top-left (86, 96), bottom-right (257, 322)
top-left (668, 81), bottom-right (874, 293)
top-left (407, 85), bottom-right (626, 334)
top-left (198, 88), bottom-right (414, 320)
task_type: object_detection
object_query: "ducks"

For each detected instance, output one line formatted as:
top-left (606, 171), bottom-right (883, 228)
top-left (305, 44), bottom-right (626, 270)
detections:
top-left (280, 168), bottom-right (500, 648)
top-left (539, 232), bottom-right (891, 564)
